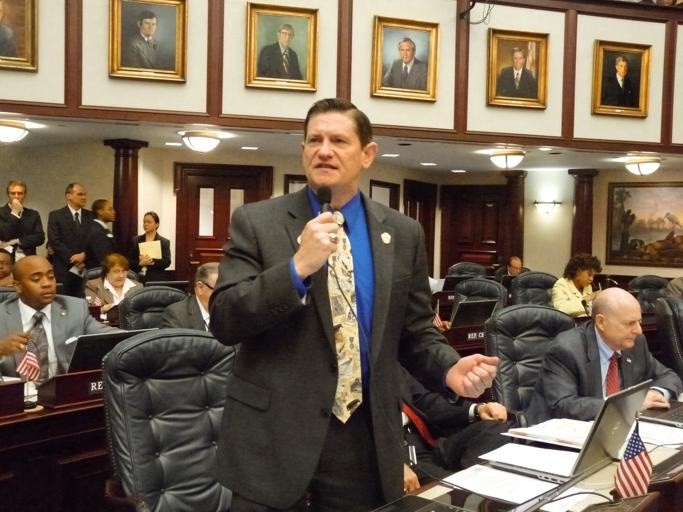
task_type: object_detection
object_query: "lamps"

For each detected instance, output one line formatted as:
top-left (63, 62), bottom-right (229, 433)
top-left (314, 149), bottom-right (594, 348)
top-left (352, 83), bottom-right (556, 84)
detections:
top-left (182, 132), bottom-right (221, 153)
top-left (624, 159), bottom-right (660, 176)
top-left (488, 151), bottom-right (526, 170)
top-left (534, 201), bottom-right (562, 216)
top-left (0, 120), bottom-right (30, 144)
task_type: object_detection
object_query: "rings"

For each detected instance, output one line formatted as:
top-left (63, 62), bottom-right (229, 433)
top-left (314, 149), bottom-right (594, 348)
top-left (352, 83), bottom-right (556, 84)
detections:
top-left (330, 233), bottom-right (338, 241)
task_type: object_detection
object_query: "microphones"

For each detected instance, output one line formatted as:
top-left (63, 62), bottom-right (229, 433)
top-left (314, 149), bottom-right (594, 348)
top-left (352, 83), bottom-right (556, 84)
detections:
top-left (581, 299), bottom-right (591, 317)
top-left (317, 184), bottom-right (331, 213)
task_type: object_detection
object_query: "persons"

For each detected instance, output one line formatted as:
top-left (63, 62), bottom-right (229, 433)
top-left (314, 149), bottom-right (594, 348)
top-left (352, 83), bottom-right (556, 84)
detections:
top-left (382, 38), bottom-right (428, 90)
top-left (433, 313), bottom-right (452, 333)
top-left (396, 361), bottom-right (515, 496)
top-left (496, 46), bottom-right (536, 98)
top-left (497, 255), bottom-right (522, 284)
top-left (1, 181), bottom-right (144, 319)
top-left (1, 3), bottom-right (18, 56)
top-left (602, 54), bottom-right (636, 106)
top-left (125, 12), bottom-right (163, 66)
top-left (209, 100), bottom-right (501, 510)
top-left (256, 25), bottom-right (300, 77)
top-left (548, 253), bottom-right (602, 321)
top-left (127, 211), bottom-right (171, 282)
top-left (526, 286), bottom-right (683, 430)
top-left (159, 261), bottom-right (220, 331)
top-left (1, 255), bottom-right (128, 386)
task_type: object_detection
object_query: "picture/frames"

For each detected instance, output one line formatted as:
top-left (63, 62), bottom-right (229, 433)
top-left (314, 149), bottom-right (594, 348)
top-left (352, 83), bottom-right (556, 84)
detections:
top-left (487, 28), bottom-right (550, 109)
top-left (0, 0), bottom-right (40, 73)
top-left (370, 15), bottom-right (441, 104)
top-left (108, 0), bottom-right (188, 84)
top-left (243, 1), bottom-right (320, 92)
top-left (605, 181), bottom-right (682, 269)
top-left (592, 39), bottom-right (652, 119)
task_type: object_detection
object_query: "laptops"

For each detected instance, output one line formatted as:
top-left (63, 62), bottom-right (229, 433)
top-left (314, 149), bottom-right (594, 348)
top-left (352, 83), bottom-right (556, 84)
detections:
top-left (442, 275), bottom-right (473, 291)
top-left (448, 299), bottom-right (499, 329)
top-left (641, 400), bottom-right (683, 429)
top-left (501, 275), bottom-right (517, 290)
top-left (371, 494), bottom-right (474, 512)
top-left (491, 379), bottom-right (654, 483)
top-left (628, 291), bottom-right (639, 301)
top-left (144, 280), bottom-right (190, 294)
top-left (65, 329), bottom-right (160, 373)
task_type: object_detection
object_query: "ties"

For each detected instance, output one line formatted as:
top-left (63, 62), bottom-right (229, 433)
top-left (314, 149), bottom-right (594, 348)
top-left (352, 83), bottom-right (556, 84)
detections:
top-left (606, 354), bottom-right (622, 396)
top-left (328, 211), bottom-right (363, 426)
top-left (283, 50), bottom-right (288, 73)
top-left (402, 66), bottom-right (408, 84)
top-left (400, 399), bottom-right (437, 447)
top-left (74, 212), bottom-right (80, 232)
top-left (515, 73), bottom-right (519, 89)
top-left (26, 313), bottom-right (49, 383)
top-left (619, 77), bottom-right (624, 89)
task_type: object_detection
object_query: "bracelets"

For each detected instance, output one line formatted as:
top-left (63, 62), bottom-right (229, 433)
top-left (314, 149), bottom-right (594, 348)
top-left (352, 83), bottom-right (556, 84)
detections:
top-left (474, 402), bottom-right (486, 418)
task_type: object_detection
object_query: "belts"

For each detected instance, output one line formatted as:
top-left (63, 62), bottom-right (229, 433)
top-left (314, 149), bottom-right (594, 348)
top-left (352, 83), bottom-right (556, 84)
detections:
top-left (403, 424), bottom-right (418, 435)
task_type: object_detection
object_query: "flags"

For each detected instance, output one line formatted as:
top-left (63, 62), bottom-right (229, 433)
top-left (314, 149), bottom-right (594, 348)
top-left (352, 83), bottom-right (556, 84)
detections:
top-left (16, 339), bottom-right (40, 382)
top-left (614, 424), bottom-right (654, 499)
